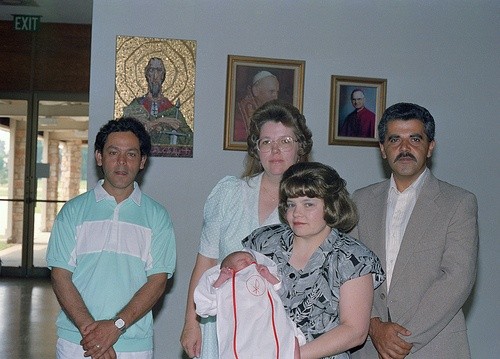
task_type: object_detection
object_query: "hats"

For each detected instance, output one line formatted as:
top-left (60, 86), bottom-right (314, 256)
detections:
top-left (251, 70), bottom-right (274, 85)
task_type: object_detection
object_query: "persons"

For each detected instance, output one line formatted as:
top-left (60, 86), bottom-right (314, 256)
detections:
top-left (346, 102), bottom-right (478, 359)
top-left (180, 98), bottom-right (313, 359)
top-left (241, 162), bottom-right (386, 359)
top-left (338, 88), bottom-right (375, 139)
top-left (46, 116), bottom-right (177, 359)
top-left (193, 248), bottom-right (307, 359)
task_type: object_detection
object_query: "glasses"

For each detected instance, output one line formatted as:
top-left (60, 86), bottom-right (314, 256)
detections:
top-left (254, 135), bottom-right (302, 152)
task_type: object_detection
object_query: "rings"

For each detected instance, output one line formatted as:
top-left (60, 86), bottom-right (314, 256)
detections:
top-left (96, 344), bottom-right (102, 350)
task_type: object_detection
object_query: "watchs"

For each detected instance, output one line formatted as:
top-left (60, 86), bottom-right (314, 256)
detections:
top-left (111, 316), bottom-right (127, 334)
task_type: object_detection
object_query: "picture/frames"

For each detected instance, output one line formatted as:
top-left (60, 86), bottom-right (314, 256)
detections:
top-left (223, 55), bottom-right (305, 151)
top-left (329, 74), bottom-right (388, 147)
